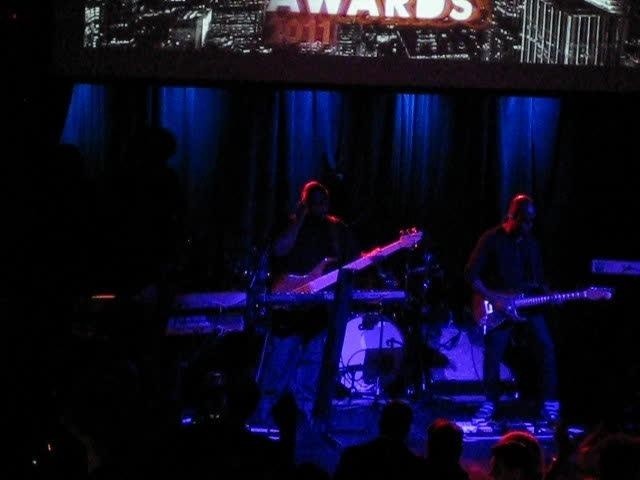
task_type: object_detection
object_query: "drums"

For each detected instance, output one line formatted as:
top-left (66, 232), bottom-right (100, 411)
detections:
top-left (330, 310), bottom-right (405, 397)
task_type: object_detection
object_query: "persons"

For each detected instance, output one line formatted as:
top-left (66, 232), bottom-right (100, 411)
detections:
top-left (464, 192), bottom-right (562, 426)
top-left (271, 179), bottom-right (352, 396)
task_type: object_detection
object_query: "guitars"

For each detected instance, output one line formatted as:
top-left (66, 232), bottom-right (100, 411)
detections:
top-left (471, 285), bottom-right (614, 329)
top-left (271, 227), bottom-right (424, 310)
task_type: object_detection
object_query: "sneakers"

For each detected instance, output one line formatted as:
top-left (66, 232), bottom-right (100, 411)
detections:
top-left (542, 398), bottom-right (561, 424)
top-left (471, 401), bottom-right (499, 425)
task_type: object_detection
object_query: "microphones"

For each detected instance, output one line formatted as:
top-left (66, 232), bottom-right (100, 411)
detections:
top-left (325, 212), bottom-right (344, 226)
